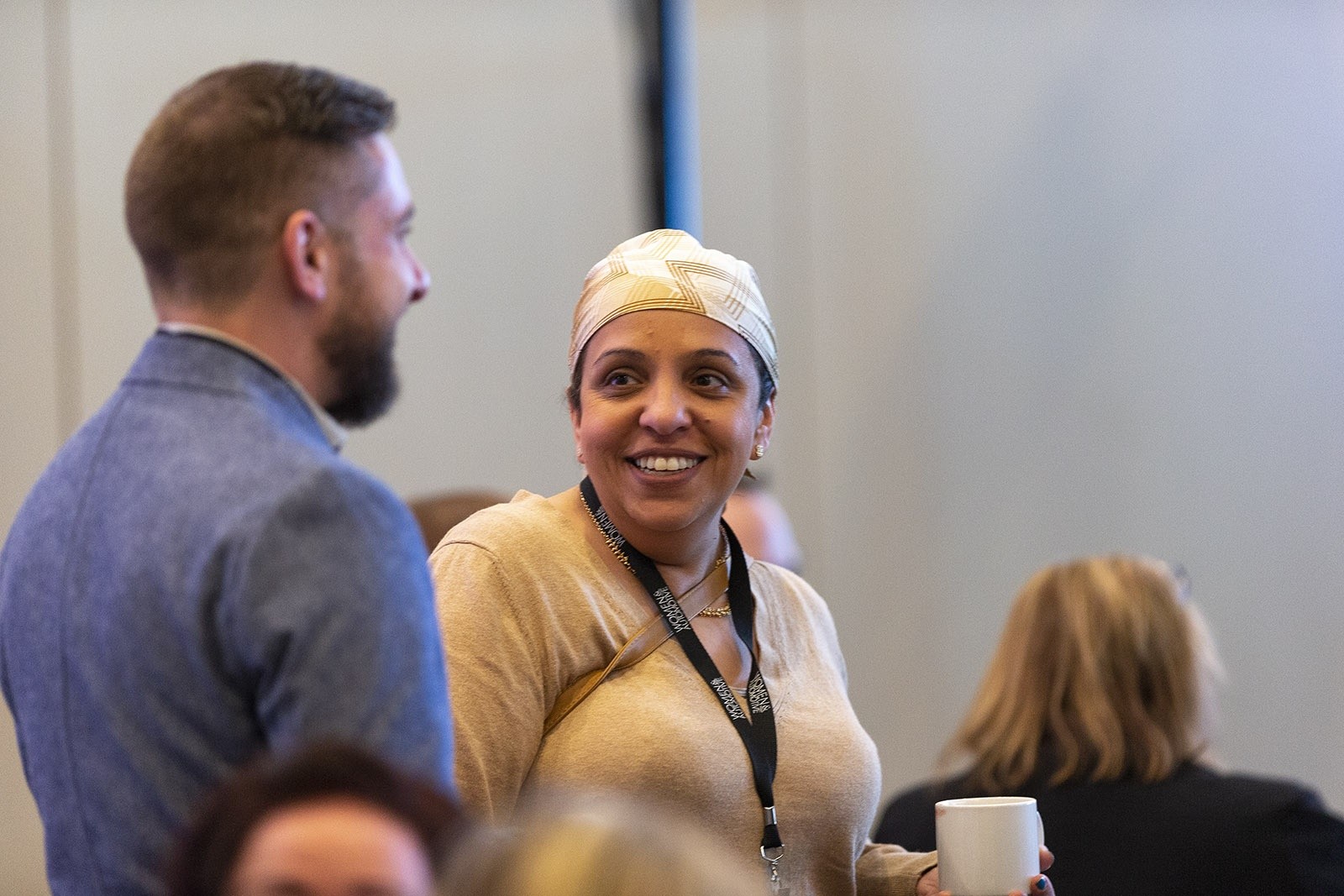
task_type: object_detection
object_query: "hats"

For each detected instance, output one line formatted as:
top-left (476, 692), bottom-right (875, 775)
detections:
top-left (567, 228), bottom-right (779, 399)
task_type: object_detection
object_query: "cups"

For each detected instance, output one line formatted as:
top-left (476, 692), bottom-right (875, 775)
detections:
top-left (935, 795), bottom-right (1045, 896)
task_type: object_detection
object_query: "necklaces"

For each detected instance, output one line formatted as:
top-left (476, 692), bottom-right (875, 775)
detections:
top-left (579, 491), bottom-right (732, 616)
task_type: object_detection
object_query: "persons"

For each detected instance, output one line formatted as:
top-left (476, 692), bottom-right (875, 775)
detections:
top-left (873, 554), bottom-right (1344, 896)
top-left (428, 229), bottom-right (1056, 896)
top-left (176, 752), bottom-right (467, 896)
top-left (0, 63), bottom-right (460, 896)
